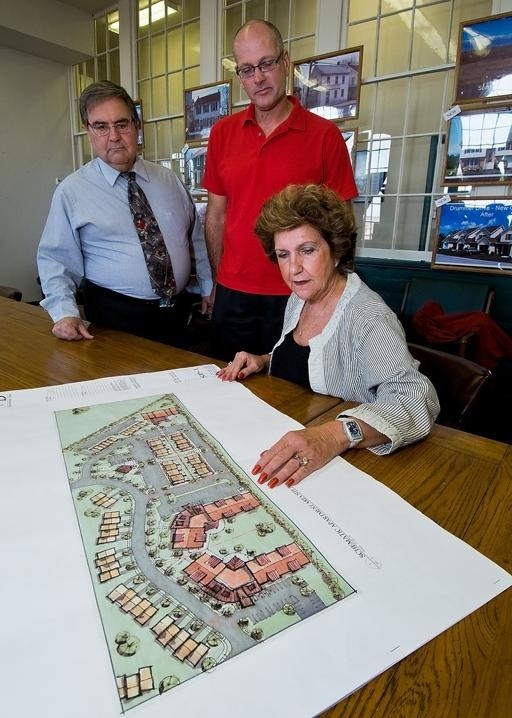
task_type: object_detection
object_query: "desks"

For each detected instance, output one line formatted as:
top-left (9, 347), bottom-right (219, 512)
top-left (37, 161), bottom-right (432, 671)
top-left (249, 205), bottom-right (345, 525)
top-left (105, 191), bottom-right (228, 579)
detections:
top-left (1, 297), bottom-right (512, 715)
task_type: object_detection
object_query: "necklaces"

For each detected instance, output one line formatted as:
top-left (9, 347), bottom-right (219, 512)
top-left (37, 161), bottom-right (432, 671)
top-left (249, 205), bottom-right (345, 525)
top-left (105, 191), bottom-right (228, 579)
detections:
top-left (300, 289), bottom-right (335, 334)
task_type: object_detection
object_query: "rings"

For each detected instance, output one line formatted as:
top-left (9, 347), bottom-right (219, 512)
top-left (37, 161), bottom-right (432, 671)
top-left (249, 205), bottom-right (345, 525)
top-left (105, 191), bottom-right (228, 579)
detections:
top-left (227, 361), bottom-right (233, 366)
top-left (293, 455), bottom-right (309, 465)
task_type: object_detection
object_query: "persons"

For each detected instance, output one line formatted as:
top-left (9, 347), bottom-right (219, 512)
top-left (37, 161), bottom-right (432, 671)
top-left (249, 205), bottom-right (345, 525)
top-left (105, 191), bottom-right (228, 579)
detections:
top-left (217, 182), bottom-right (442, 488)
top-left (199, 18), bottom-right (360, 363)
top-left (36, 80), bottom-right (214, 351)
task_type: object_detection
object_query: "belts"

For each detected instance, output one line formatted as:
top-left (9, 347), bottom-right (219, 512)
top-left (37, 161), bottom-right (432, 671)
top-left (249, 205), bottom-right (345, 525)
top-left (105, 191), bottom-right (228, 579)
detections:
top-left (146, 297), bottom-right (175, 307)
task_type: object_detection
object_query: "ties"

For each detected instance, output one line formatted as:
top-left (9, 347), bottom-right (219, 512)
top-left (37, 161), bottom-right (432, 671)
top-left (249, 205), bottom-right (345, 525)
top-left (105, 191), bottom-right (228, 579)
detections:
top-left (119, 171), bottom-right (176, 298)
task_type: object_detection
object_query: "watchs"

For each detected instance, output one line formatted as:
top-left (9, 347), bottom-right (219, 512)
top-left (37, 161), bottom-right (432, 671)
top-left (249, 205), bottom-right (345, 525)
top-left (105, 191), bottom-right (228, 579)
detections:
top-left (336, 417), bottom-right (365, 448)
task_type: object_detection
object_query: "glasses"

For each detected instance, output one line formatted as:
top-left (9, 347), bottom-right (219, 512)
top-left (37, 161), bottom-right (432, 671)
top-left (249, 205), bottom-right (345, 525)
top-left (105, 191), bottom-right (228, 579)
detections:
top-left (88, 120), bottom-right (133, 136)
top-left (235, 50), bottom-right (282, 79)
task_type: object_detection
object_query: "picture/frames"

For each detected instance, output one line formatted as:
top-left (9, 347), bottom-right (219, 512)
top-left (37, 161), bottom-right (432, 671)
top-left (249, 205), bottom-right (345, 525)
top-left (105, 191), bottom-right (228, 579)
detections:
top-left (179, 43), bottom-right (366, 211)
top-left (430, 11), bottom-right (512, 278)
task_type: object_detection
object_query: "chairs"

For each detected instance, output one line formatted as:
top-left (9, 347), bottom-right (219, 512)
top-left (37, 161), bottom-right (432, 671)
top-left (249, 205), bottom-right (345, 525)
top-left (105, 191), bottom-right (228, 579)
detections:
top-left (405, 322), bottom-right (492, 426)
